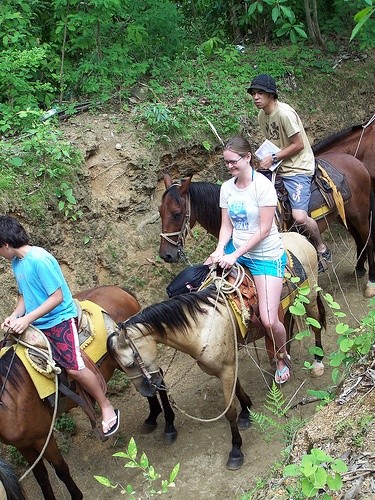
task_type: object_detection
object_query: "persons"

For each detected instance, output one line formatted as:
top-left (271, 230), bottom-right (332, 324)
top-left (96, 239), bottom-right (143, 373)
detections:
top-left (246, 75), bottom-right (332, 262)
top-left (0, 215), bottom-right (120, 436)
top-left (203, 136), bottom-right (290, 384)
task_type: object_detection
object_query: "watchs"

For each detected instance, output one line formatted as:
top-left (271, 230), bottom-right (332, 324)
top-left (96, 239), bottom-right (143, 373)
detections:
top-left (271, 153), bottom-right (278, 165)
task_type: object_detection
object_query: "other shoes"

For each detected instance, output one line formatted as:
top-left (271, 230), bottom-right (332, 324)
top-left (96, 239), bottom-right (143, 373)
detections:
top-left (319, 243), bottom-right (331, 261)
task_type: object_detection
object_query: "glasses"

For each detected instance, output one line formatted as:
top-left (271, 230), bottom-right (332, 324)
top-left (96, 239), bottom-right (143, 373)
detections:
top-left (223, 153), bottom-right (248, 166)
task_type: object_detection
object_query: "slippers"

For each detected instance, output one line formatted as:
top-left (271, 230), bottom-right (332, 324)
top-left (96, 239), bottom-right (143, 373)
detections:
top-left (102, 408), bottom-right (121, 436)
top-left (274, 354), bottom-right (290, 384)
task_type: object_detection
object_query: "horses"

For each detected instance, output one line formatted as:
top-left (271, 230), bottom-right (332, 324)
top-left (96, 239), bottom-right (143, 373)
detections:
top-left (0, 284), bottom-right (178, 500)
top-left (101, 231), bottom-right (326, 476)
top-left (159, 115), bottom-right (375, 299)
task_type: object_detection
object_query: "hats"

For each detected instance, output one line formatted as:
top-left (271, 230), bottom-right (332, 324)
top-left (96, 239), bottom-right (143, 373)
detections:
top-left (247, 74), bottom-right (279, 99)
